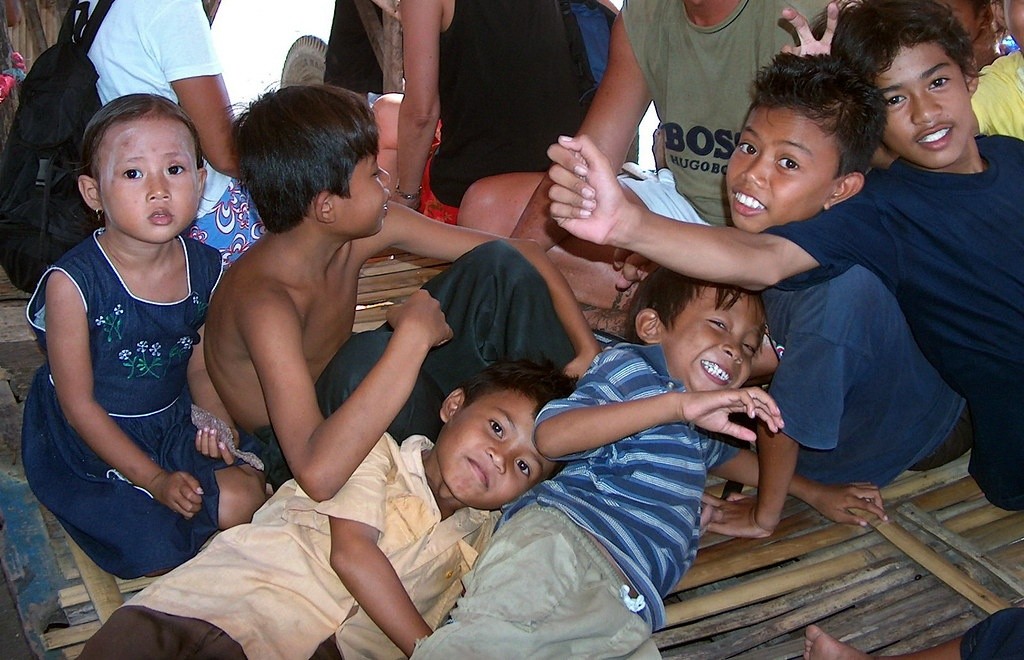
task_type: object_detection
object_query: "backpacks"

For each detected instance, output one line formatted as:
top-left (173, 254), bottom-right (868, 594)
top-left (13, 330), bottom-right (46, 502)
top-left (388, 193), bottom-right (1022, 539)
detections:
top-left (0, 0), bottom-right (114, 294)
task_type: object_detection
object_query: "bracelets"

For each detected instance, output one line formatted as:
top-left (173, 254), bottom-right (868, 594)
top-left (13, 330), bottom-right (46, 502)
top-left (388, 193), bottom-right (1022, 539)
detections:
top-left (395, 184), bottom-right (421, 200)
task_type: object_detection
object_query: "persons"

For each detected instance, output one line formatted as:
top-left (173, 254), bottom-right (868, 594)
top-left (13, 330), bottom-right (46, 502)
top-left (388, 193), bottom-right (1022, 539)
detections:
top-left (73, 356), bottom-right (578, 660)
top-left (547, 0), bottom-right (1024, 513)
top-left (413, 266), bottom-right (889, 660)
top-left (457, 0), bottom-right (834, 309)
top-left (19, 93), bottom-right (266, 582)
top-left (203, 83), bottom-right (603, 502)
top-left (371, 0), bottom-right (619, 245)
top-left (804, 607), bottom-right (1024, 660)
top-left (708, 59), bottom-right (971, 540)
top-left (71, 0), bottom-right (270, 270)
